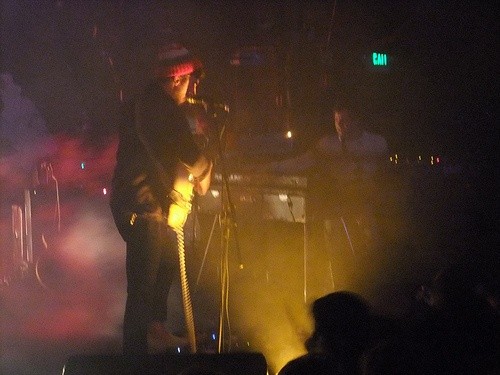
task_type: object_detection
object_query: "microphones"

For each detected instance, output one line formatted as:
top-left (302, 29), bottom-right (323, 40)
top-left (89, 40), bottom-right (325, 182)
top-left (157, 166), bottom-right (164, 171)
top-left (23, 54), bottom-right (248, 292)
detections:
top-left (187, 95), bottom-right (227, 108)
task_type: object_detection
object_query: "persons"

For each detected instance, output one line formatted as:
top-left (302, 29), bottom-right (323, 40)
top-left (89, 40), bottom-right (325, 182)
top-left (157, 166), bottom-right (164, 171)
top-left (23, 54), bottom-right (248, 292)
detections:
top-left (275, 258), bottom-right (500, 375)
top-left (108, 38), bottom-right (209, 356)
top-left (235, 101), bottom-right (390, 293)
top-left (148, 54), bottom-right (214, 354)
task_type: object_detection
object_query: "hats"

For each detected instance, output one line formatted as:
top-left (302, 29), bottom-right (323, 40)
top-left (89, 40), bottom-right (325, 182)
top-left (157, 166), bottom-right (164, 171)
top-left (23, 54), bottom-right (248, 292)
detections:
top-left (152, 41), bottom-right (202, 78)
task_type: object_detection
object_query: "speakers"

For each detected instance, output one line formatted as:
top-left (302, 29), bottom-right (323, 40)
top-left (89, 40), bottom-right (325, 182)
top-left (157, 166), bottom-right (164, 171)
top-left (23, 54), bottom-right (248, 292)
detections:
top-left (62, 351), bottom-right (270, 375)
top-left (192, 181), bottom-right (307, 375)
